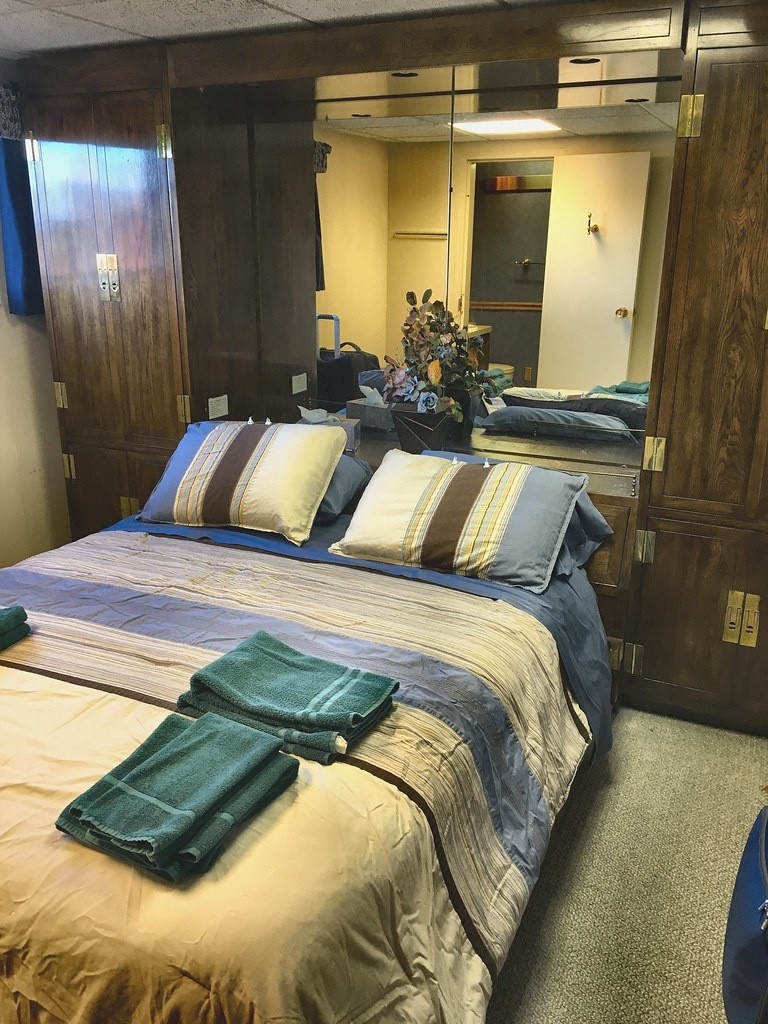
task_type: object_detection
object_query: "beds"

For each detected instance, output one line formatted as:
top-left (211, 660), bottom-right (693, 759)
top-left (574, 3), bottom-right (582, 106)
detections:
top-left (0, 511), bottom-right (613, 1024)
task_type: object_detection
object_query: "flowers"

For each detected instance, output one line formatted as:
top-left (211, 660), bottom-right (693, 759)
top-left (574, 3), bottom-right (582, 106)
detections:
top-left (419, 289), bottom-right (485, 389)
top-left (382, 291), bottom-right (478, 423)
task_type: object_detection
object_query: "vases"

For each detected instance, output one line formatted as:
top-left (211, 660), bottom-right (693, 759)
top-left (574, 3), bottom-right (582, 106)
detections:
top-left (445, 384), bottom-right (484, 435)
top-left (389, 384), bottom-right (470, 454)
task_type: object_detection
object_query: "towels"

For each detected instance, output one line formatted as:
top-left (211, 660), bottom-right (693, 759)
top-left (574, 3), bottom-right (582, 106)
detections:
top-left (174, 629), bottom-right (400, 766)
top-left (53, 713), bottom-right (301, 880)
top-left (587, 380), bottom-right (650, 404)
top-left (479, 368), bottom-right (514, 400)
top-left (0, 603), bottom-right (31, 651)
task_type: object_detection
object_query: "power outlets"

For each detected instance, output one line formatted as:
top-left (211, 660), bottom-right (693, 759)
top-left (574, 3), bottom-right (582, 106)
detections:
top-left (523, 367), bottom-right (532, 381)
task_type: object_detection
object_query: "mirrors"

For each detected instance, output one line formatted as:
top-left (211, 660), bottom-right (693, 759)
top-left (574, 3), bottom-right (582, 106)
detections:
top-left (168, 48), bottom-right (673, 466)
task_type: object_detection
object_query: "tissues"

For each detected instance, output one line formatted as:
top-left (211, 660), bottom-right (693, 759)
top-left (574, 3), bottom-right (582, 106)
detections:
top-left (293, 404), bottom-right (361, 452)
top-left (345, 385), bottom-right (398, 430)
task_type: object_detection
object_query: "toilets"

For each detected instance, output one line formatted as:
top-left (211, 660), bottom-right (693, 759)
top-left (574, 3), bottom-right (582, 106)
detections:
top-left (488, 363), bottom-right (514, 383)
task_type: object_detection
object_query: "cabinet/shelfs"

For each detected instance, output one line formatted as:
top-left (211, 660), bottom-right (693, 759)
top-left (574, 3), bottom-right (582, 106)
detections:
top-left (246, 75), bottom-right (320, 398)
top-left (618, 0), bottom-right (768, 738)
top-left (22, 89), bottom-right (260, 542)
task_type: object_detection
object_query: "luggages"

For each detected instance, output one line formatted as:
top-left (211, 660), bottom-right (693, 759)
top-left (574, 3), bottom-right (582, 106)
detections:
top-left (317, 313), bottom-right (382, 413)
top-left (722, 805), bottom-right (768, 1024)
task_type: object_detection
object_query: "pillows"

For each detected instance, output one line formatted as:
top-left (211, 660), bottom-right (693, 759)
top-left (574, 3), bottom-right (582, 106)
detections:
top-left (328, 446), bottom-right (590, 595)
top-left (420, 449), bottom-right (614, 578)
top-left (358, 370), bottom-right (485, 428)
top-left (314, 455), bottom-right (374, 526)
top-left (135, 421), bottom-right (347, 547)
top-left (499, 386), bottom-right (648, 440)
top-left (480, 405), bottom-right (639, 445)
